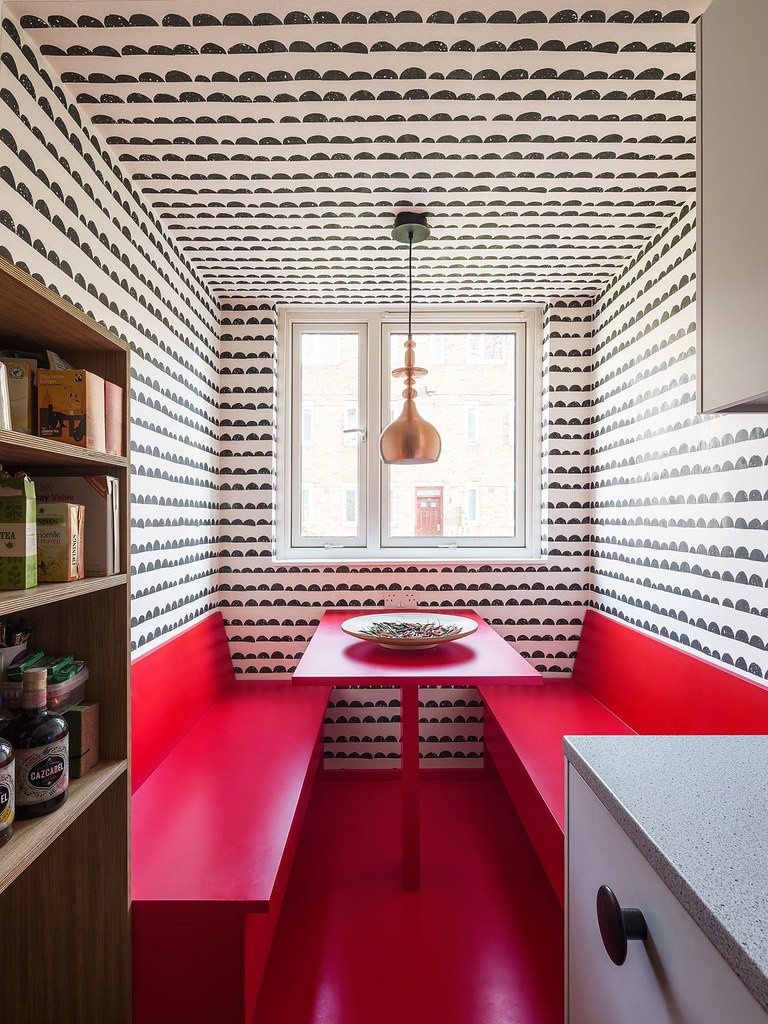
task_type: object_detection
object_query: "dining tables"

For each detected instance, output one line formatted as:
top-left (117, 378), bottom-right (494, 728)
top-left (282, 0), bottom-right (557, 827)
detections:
top-left (292, 611), bottom-right (543, 887)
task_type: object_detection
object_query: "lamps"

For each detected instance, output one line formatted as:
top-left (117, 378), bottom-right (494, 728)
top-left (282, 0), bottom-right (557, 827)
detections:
top-left (377, 212), bottom-right (445, 466)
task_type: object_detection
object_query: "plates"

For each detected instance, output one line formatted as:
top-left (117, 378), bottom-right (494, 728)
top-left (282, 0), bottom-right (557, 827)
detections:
top-left (341, 613), bottom-right (478, 650)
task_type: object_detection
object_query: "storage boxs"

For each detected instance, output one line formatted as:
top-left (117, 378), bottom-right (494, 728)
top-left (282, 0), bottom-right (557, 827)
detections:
top-left (64, 699), bottom-right (102, 778)
top-left (0, 470), bottom-right (122, 588)
top-left (0, 355), bottom-right (126, 458)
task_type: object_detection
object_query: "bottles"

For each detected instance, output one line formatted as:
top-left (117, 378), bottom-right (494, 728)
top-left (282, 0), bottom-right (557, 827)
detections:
top-left (6, 667), bottom-right (70, 822)
top-left (0, 737), bottom-right (15, 845)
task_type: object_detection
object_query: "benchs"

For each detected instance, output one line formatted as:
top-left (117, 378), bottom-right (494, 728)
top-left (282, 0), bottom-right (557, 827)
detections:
top-left (477, 609), bottom-right (768, 910)
top-left (131, 614), bottom-right (334, 1024)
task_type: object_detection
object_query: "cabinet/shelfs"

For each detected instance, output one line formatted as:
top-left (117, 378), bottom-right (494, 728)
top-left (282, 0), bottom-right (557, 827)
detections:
top-left (561, 760), bottom-right (768, 1023)
top-left (0, 255), bottom-right (134, 1024)
top-left (697, 2), bottom-right (768, 413)
